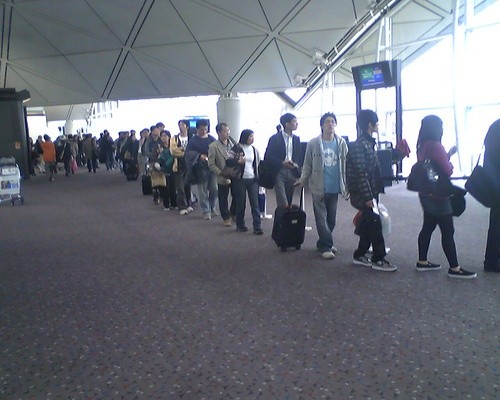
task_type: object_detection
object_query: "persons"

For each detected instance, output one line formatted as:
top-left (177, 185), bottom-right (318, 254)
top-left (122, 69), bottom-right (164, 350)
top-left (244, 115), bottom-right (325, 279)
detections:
top-left (226, 129), bottom-right (263, 235)
top-left (99, 130), bottom-right (115, 173)
top-left (294, 113), bottom-right (348, 259)
top-left (28, 122), bottom-right (181, 211)
top-left (208, 123), bottom-right (238, 226)
top-left (345, 110), bottom-right (397, 271)
top-left (184, 119), bottom-right (219, 220)
top-left (170, 120), bottom-right (193, 216)
top-left (484, 119), bottom-right (500, 272)
top-left (276, 125), bottom-right (282, 134)
top-left (266, 113), bottom-right (301, 207)
top-left (416, 116), bottom-right (477, 279)
top-left (40, 134), bottom-right (55, 182)
top-left (83, 135), bottom-right (96, 174)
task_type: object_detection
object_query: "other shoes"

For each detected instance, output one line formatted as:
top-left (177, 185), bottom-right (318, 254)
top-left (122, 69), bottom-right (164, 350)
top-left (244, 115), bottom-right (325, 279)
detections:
top-left (180, 205), bottom-right (194, 215)
top-left (254, 229), bottom-right (263, 235)
top-left (321, 251), bottom-right (335, 259)
top-left (447, 267), bottom-right (477, 278)
top-left (212, 208), bottom-right (218, 216)
top-left (224, 216), bottom-right (231, 226)
top-left (174, 207), bottom-right (178, 210)
top-left (416, 260), bottom-right (441, 271)
top-left (372, 260), bottom-right (397, 271)
top-left (353, 255), bottom-right (372, 266)
top-left (154, 199), bottom-right (159, 204)
top-left (317, 241), bottom-right (337, 252)
top-left (202, 211), bottom-right (211, 219)
top-left (237, 226), bottom-right (248, 232)
top-left (165, 207), bottom-right (170, 211)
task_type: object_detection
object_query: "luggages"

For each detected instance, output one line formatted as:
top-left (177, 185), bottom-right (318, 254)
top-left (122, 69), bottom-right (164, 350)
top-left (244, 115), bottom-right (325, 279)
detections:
top-left (142, 171), bottom-right (153, 195)
top-left (271, 185), bottom-right (306, 251)
top-left (127, 155), bottom-right (139, 180)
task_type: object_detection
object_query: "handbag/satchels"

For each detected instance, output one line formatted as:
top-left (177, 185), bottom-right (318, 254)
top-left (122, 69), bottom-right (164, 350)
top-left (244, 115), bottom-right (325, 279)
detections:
top-left (58, 150), bottom-right (64, 161)
top-left (258, 160), bottom-right (279, 189)
top-left (464, 165), bottom-right (500, 208)
top-left (354, 210), bottom-right (381, 242)
top-left (406, 158), bottom-right (451, 195)
top-left (193, 162), bottom-right (212, 184)
top-left (178, 157), bottom-right (186, 174)
top-left (71, 159), bottom-right (78, 173)
top-left (450, 182), bottom-right (467, 216)
top-left (258, 186), bottom-right (267, 217)
top-left (352, 209), bottom-right (363, 224)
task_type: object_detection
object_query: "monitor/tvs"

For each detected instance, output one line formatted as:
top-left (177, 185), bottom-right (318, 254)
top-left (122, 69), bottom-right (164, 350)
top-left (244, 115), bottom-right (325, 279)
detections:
top-left (351, 61), bottom-right (394, 90)
